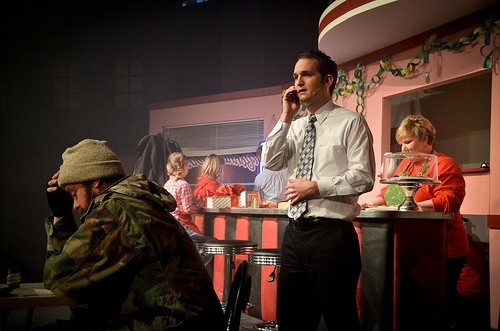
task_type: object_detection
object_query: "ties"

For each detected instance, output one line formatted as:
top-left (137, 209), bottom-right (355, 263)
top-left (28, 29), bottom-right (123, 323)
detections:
top-left (290, 114), bottom-right (316, 220)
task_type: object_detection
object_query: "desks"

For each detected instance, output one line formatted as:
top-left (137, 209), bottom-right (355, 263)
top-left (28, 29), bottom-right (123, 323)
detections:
top-left (193, 207), bottom-right (454, 331)
top-left (0, 283), bottom-right (77, 331)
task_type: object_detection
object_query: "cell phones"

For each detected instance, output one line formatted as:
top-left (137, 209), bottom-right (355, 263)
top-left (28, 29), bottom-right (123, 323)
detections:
top-left (289, 92), bottom-right (297, 102)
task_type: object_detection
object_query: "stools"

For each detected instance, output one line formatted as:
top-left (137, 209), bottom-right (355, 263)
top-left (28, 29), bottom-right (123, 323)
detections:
top-left (249, 248), bottom-right (282, 331)
top-left (191, 235), bottom-right (218, 266)
top-left (203, 239), bottom-right (259, 313)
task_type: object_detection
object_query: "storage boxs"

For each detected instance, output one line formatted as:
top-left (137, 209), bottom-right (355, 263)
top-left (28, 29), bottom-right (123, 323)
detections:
top-left (207, 196), bottom-right (231, 208)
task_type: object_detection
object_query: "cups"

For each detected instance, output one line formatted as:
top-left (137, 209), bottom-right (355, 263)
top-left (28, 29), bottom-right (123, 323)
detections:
top-left (7, 269), bottom-right (21, 288)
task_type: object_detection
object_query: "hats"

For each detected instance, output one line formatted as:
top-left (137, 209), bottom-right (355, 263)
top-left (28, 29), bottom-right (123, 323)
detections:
top-left (58, 139), bottom-right (126, 186)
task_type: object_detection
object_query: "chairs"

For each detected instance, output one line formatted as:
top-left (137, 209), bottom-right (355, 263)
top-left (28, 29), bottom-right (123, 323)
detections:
top-left (225, 260), bottom-right (252, 331)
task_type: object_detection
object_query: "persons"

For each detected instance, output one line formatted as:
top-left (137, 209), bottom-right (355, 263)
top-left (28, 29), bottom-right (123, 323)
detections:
top-left (261, 49), bottom-right (376, 331)
top-left (253, 145), bottom-right (288, 205)
top-left (164, 151), bottom-right (225, 265)
top-left (360, 114), bottom-right (470, 331)
top-left (43, 138), bottom-right (227, 331)
